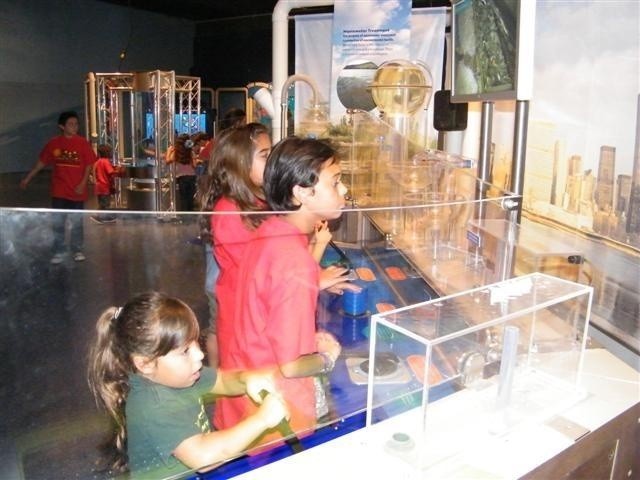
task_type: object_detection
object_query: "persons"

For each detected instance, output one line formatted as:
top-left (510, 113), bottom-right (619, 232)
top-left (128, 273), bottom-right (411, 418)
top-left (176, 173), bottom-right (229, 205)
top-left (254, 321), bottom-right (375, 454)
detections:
top-left (212, 134), bottom-right (349, 457)
top-left (166, 109), bottom-right (362, 338)
top-left (21, 111), bottom-right (97, 265)
top-left (90, 144), bottom-right (126, 224)
top-left (87, 292), bottom-right (290, 480)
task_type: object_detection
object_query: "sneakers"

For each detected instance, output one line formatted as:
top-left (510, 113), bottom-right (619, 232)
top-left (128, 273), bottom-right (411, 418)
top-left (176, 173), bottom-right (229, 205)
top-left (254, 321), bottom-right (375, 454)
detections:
top-left (49, 251), bottom-right (86, 264)
top-left (90, 215), bottom-right (117, 225)
